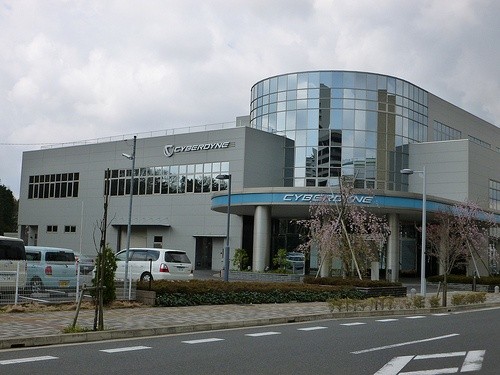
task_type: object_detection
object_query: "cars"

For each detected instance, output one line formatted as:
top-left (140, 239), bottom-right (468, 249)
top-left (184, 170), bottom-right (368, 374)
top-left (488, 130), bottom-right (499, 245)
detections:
top-left (73, 252), bottom-right (94, 274)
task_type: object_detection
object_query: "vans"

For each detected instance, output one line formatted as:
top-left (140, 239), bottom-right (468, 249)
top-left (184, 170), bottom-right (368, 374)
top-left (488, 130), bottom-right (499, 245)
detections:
top-left (92, 248), bottom-right (192, 286)
top-left (24, 246), bottom-right (76, 296)
top-left (0, 237), bottom-right (27, 291)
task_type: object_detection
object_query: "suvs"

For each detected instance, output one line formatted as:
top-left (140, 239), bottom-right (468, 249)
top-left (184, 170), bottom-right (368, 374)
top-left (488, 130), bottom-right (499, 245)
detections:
top-left (285, 253), bottom-right (304, 270)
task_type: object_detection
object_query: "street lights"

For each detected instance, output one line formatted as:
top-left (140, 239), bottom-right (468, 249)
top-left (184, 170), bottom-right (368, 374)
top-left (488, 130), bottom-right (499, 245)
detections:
top-left (120, 134), bottom-right (137, 298)
top-left (217, 174), bottom-right (232, 281)
top-left (397, 164), bottom-right (427, 297)
